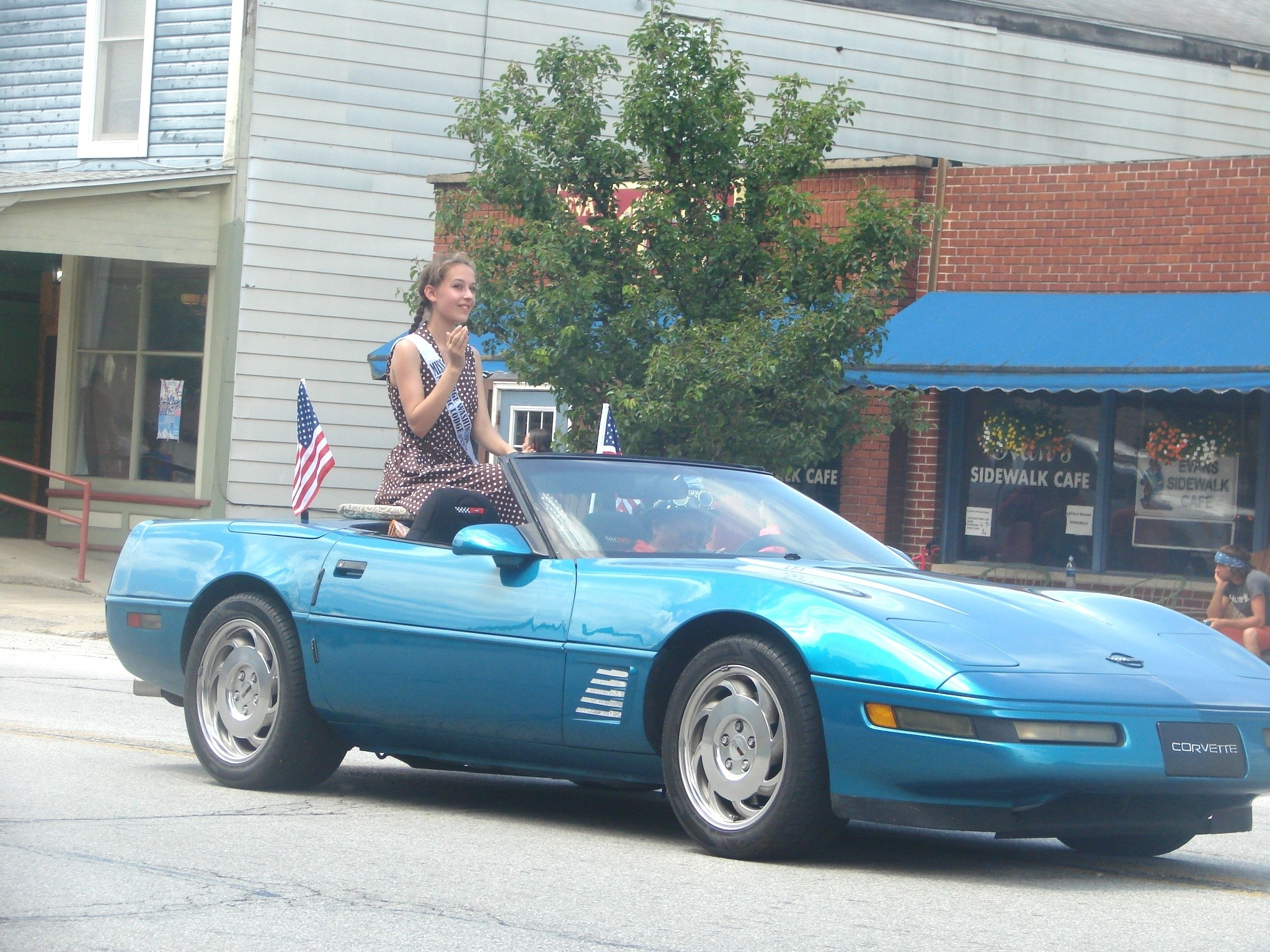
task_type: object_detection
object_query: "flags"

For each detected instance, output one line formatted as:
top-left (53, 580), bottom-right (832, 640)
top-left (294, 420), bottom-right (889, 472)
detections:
top-left (596, 403), bottom-right (641, 515)
top-left (291, 380), bottom-right (336, 516)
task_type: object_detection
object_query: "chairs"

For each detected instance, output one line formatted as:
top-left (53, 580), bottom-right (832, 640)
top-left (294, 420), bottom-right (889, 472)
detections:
top-left (403, 488), bottom-right (502, 547)
top-left (980, 562), bottom-right (1052, 588)
top-left (580, 510), bottom-right (649, 553)
top-left (1118, 574), bottom-right (1187, 610)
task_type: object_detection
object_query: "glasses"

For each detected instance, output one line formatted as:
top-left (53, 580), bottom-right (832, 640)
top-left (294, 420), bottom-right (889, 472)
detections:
top-left (665, 522), bottom-right (711, 544)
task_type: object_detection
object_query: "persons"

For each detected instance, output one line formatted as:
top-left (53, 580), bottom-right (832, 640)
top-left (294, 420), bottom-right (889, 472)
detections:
top-left (1113, 479), bottom-right (1171, 572)
top-left (631, 494), bottom-right (789, 554)
top-left (1142, 459), bottom-right (1163, 505)
top-left (997, 464), bottom-right (1093, 571)
top-left (374, 252), bottom-right (536, 526)
top-left (1203, 545), bottom-right (1270, 659)
top-left (523, 429), bottom-right (552, 454)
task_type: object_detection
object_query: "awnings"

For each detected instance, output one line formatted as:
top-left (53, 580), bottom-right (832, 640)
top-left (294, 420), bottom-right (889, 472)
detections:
top-left (369, 278), bottom-right (829, 381)
top-left (829, 292), bottom-right (1270, 394)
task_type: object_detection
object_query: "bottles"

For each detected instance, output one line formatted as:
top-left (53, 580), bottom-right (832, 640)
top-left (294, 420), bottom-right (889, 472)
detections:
top-left (1065, 556), bottom-right (1076, 588)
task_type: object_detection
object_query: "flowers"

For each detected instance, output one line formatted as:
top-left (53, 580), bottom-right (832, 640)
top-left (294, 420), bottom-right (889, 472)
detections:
top-left (975, 397), bottom-right (1076, 461)
top-left (1145, 398), bottom-right (1247, 468)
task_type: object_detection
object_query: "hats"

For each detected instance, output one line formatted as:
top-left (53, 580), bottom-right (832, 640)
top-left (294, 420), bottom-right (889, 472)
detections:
top-left (641, 474), bottom-right (734, 525)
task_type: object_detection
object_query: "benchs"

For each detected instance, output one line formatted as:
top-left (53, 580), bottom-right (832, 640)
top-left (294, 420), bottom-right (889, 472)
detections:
top-left (341, 502), bottom-right (411, 521)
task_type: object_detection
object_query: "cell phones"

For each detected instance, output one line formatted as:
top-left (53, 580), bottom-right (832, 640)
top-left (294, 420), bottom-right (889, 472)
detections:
top-left (1204, 621), bottom-right (1211, 626)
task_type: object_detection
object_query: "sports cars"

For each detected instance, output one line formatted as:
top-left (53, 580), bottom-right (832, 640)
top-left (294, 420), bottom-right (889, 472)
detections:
top-left (106, 452), bottom-right (1270, 858)
